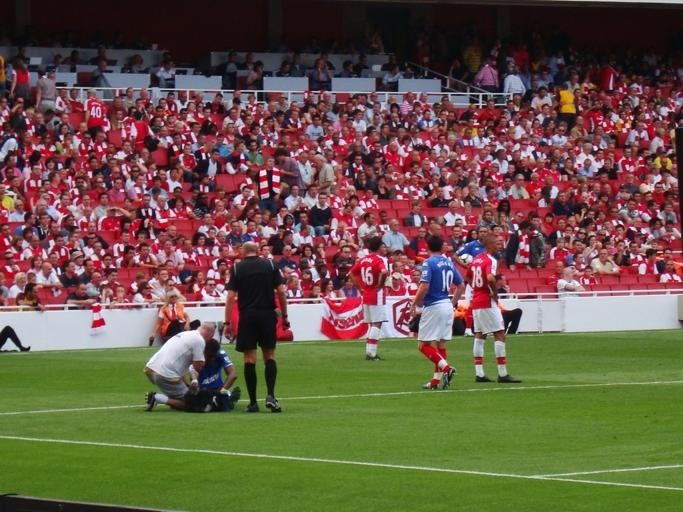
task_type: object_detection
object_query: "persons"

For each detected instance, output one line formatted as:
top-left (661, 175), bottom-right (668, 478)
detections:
top-left (0, 324), bottom-right (31, 352)
top-left (149, 290), bottom-right (190, 349)
top-left (348, 236), bottom-right (387, 361)
top-left (448, 296), bottom-right (467, 336)
top-left (223, 242), bottom-right (290, 413)
top-left (189, 338), bottom-right (240, 413)
top-left (1, 30), bottom-right (683, 313)
top-left (143, 320), bottom-right (216, 412)
top-left (498, 303), bottom-right (522, 334)
top-left (408, 234), bottom-right (466, 390)
top-left (462, 234), bottom-right (522, 384)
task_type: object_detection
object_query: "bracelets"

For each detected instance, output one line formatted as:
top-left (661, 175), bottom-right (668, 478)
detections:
top-left (191, 379), bottom-right (199, 386)
top-left (222, 319), bottom-right (232, 325)
top-left (282, 314), bottom-right (288, 318)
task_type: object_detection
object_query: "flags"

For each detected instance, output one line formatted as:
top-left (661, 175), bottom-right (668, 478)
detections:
top-left (319, 296), bottom-right (371, 339)
top-left (230, 297), bottom-right (294, 342)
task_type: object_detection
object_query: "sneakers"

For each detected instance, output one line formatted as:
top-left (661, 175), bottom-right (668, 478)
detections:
top-left (422, 366), bottom-right (521, 389)
top-left (143, 386), bottom-right (282, 414)
top-left (365, 354), bottom-right (382, 360)
